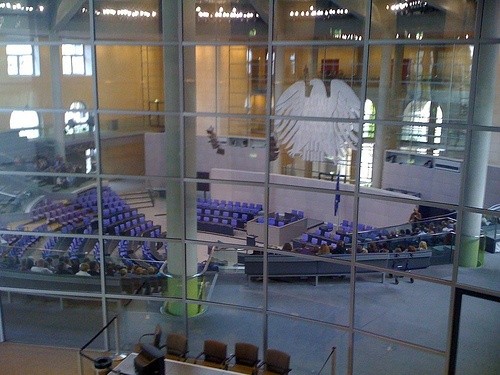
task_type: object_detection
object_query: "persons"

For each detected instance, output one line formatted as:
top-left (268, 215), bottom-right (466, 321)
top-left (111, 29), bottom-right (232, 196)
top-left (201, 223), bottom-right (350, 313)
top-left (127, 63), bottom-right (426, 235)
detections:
top-left (283, 217), bottom-right (499, 260)
top-left (31, 255), bottom-right (159, 275)
top-left (7, 151), bottom-right (88, 190)
top-left (409, 208), bottom-right (421, 232)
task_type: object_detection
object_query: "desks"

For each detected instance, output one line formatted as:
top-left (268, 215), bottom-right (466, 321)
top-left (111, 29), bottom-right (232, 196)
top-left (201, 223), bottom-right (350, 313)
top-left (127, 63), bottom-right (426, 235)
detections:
top-left (107, 352), bottom-right (248, 375)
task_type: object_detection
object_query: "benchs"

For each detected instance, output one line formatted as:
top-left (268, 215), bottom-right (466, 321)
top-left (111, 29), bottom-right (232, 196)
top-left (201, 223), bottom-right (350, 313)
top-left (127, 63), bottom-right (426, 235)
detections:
top-left (243, 250), bottom-right (433, 286)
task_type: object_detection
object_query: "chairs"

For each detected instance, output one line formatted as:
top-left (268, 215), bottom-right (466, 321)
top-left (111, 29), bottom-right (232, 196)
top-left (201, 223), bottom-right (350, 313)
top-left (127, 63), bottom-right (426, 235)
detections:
top-left (2, 184), bottom-right (166, 274)
top-left (194, 339), bottom-right (228, 370)
top-left (229, 342), bottom-right (261, 375)
top-left (197, 198), bottom-right (304, 231)
top-left (257, 349), bottom-right (293, 375)
top-left (159, 333), bottom-right (190, 362)
top-left (135, 325), bottom-right (161, 353)
top-left (293, 220), bottom-right (435, 254)
